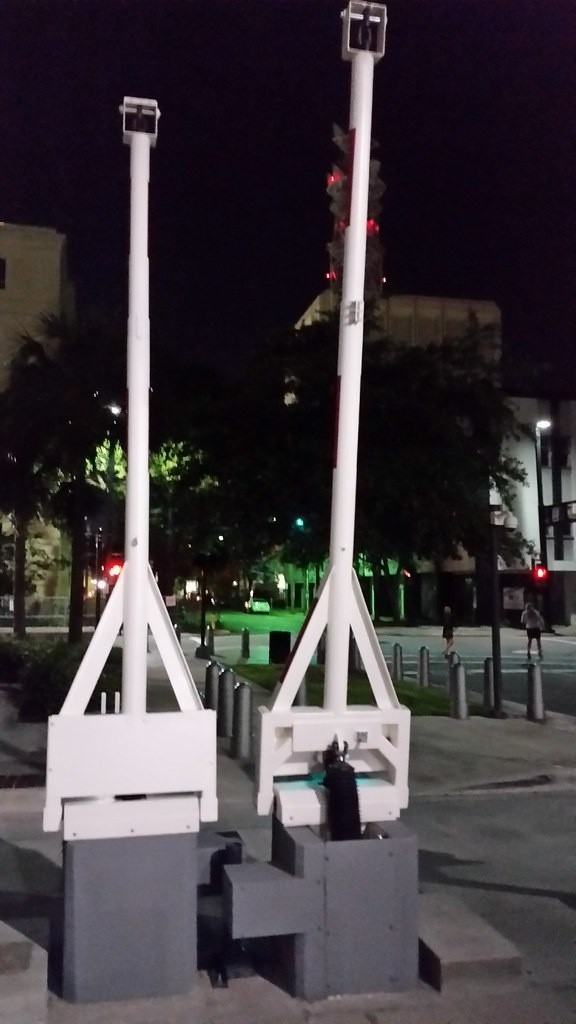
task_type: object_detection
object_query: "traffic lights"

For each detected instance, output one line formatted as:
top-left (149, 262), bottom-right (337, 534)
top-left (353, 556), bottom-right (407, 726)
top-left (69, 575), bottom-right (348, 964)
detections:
top-left (108, 560), bottom-right (121, 584)
top-left (536, 565), bottom-right (546, 579)
top-left (293, 519), bottom-right (309, 534)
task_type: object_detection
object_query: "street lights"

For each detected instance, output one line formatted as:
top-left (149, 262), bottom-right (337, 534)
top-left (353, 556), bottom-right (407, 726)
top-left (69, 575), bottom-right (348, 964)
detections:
top-left (118, 95), bottom-right (163, 715)
top-left (323, 2), bottom-right (391, 710)
top-left (531, 416), bottom-right (552, 634)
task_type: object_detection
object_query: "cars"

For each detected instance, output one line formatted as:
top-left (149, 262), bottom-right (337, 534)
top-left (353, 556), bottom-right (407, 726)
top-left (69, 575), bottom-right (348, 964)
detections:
top-left (244, 598), bottom-right (270, 614)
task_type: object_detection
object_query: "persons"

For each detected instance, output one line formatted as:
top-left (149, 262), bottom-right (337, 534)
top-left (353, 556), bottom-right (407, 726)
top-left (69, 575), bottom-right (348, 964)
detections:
top-left (521, 602), bottom-right (546, 658)
top-left (441, 606), bottom-right (455, 657)
